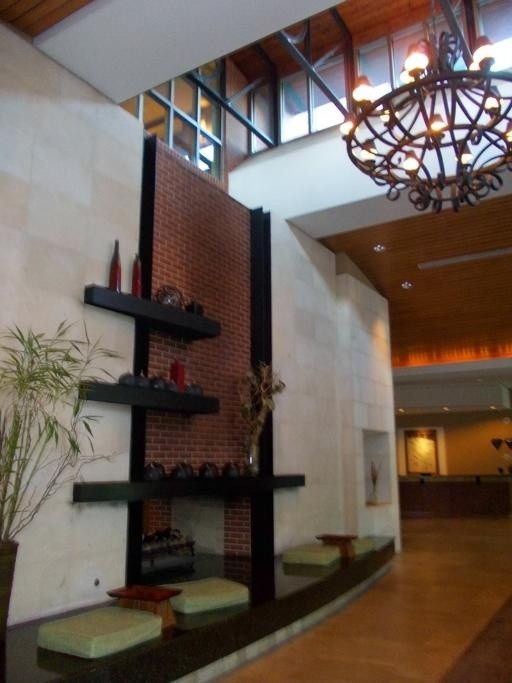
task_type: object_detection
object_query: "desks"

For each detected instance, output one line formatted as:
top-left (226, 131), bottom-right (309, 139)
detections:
top-left (106, 584), bottom-right (183, 628)
top-left (315, 533), bottom-right (358, 562)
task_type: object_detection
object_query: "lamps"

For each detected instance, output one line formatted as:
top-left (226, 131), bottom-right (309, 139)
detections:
top-left (339, 1), bottom-right (512, 213)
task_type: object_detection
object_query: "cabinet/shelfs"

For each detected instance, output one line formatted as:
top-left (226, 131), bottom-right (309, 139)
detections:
top-left (78, 380), bottom-right (221, 416)
top-left (72, 473), bottom-right (306, 504)
top-left (83, 284), bottom-right (222, 342)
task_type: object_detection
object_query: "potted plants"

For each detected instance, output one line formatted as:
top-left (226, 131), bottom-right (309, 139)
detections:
top-left (233, 360), bottom-right (285, 475)
top-left (0, 314), bottom-right (119, 657)
